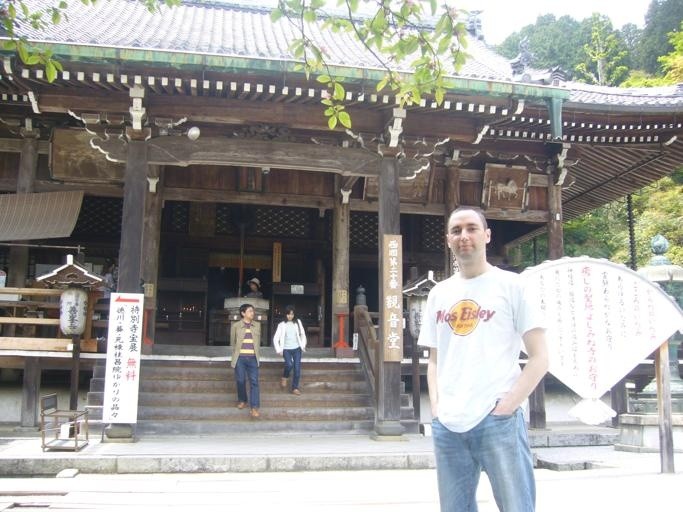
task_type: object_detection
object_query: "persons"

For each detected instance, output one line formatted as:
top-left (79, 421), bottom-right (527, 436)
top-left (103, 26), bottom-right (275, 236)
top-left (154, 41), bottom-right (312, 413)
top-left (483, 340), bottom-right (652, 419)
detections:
top-left (273, 305), bottom-right (307, 395)
top-left (96, 261), bottom-right (115, 338)
top-left (230, 304), bottom-right (261, 417)
top-left (417, 207), bottom-right (549, 512)
top-left (246, 277), bottom-right (263, 297)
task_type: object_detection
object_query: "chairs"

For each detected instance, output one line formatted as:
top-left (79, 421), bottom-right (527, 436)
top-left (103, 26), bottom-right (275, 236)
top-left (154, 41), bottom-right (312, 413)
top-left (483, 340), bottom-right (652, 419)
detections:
top-left (38, 392), bottom-right (89, 451)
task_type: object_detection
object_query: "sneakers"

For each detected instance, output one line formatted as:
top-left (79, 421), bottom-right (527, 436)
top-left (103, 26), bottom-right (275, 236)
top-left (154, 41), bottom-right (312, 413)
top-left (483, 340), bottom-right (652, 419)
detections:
top-left (281, 377), bottom-right (288, 388)
top-left (237, 401), bottom-right (247, 409)
top-left (291, 389), bottom-right (302, 396)
top-left (249, 408), bottom-right (260, 419)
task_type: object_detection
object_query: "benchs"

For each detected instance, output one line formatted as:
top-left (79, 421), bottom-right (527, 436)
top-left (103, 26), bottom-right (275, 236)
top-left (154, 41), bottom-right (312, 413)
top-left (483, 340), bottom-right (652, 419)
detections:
top-left (617, 414), bottom-right (681, 453)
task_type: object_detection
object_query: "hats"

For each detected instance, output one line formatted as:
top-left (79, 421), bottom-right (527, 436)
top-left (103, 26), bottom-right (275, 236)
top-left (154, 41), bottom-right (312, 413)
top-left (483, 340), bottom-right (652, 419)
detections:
top-left (246, 277), bottom-right (260, 288)
